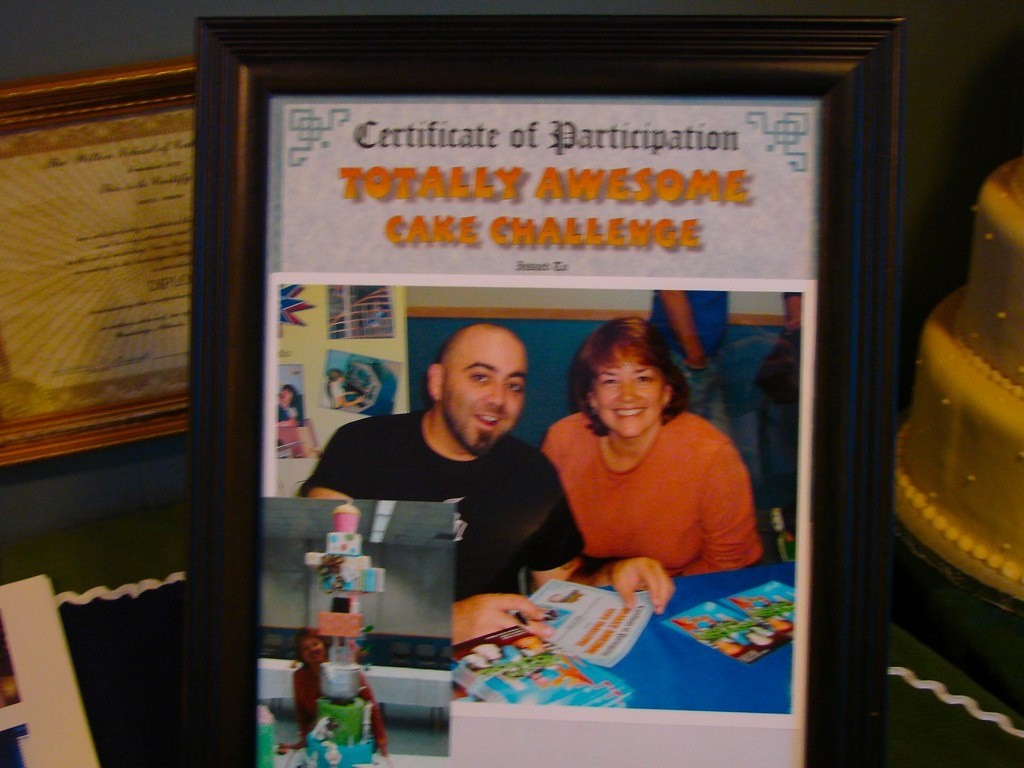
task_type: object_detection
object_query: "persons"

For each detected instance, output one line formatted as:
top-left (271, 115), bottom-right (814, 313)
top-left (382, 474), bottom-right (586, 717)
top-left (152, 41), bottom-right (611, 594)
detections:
top-left (648, 290), bottom-right (734, 439)
top-left (297, 325), bottom-right (675, 645)
top-left (327, 369), bottom-right (365, 409)
top-left (541, 318), bottom-right (762, 577)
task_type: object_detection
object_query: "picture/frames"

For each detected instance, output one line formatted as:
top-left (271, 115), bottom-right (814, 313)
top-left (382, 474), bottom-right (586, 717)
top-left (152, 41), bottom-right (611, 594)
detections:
top-left (0, 573), bottom-right (99, 768)
top-left (0, 56), bottom-right (197, 469)
top-left (194, 15), bottom-right (909, 767)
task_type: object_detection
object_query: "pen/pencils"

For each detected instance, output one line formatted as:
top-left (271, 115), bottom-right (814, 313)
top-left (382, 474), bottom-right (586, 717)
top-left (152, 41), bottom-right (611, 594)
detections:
top-left (508, 608), bottom-right (526, 625)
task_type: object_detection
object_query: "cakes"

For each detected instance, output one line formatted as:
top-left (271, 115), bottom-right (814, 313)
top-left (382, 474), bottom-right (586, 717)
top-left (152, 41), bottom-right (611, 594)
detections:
top-left (888, 157), bottom-right (1024, 607)
